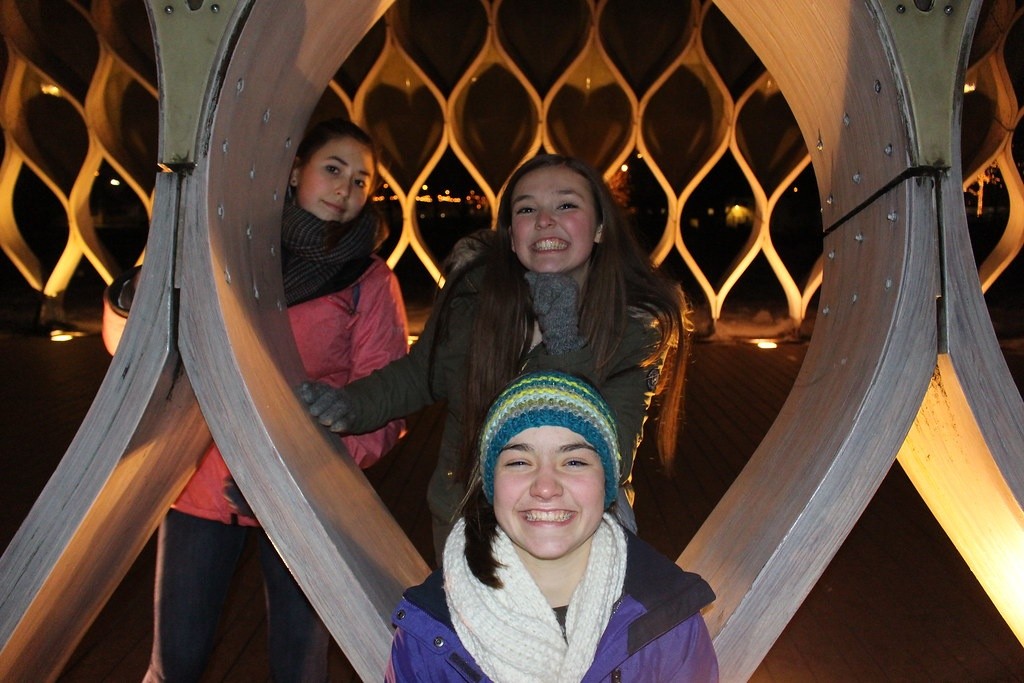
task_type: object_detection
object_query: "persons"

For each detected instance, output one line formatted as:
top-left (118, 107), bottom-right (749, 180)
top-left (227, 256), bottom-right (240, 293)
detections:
top-left (102, 118), bottom-right (413, 683)
top-left (299, 152), bottom-right (694, 579)
top-left (379, 367), bottom-right (723, 682)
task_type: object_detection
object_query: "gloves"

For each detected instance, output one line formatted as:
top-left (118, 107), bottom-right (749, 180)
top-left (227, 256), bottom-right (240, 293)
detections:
top-left (224, 477), bottom-right (255, 523)
top-left (299, 380), bottom-right (356, 437)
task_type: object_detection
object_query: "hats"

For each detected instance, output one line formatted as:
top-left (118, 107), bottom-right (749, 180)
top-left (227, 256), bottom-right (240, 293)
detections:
top-left (476, 368), bottom-right (626, 508)
